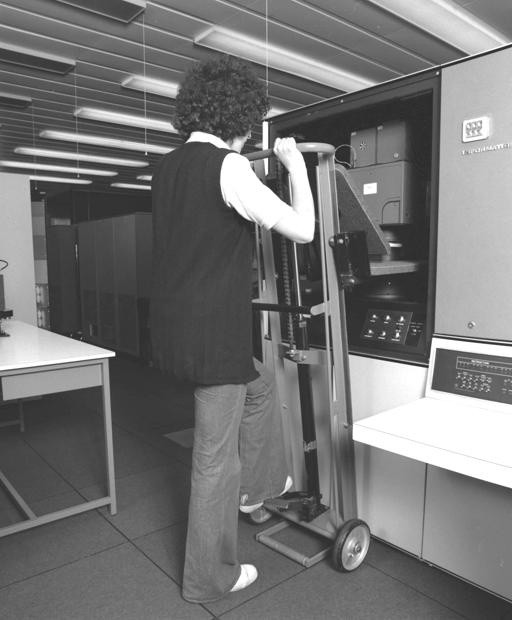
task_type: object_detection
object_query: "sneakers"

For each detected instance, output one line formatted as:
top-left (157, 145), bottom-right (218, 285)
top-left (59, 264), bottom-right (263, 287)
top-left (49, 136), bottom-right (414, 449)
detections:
top-left (239, 476), bottom-right (293, 516)
top-left (229, 564), bottom-right (258, 592)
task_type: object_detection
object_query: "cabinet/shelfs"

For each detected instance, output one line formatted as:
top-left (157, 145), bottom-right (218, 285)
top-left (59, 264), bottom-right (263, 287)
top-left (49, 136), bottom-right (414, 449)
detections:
top-left (76, 212), bottom-right (152, 357)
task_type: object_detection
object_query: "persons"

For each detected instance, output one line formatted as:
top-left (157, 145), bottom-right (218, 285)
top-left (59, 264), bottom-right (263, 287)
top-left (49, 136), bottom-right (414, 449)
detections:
top-left (145, 55), bottom-right (316, 605)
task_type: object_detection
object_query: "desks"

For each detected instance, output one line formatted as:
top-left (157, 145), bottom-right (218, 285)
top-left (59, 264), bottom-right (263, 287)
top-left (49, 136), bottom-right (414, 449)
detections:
top-left (0, 318), bottom-right (118, 538)
